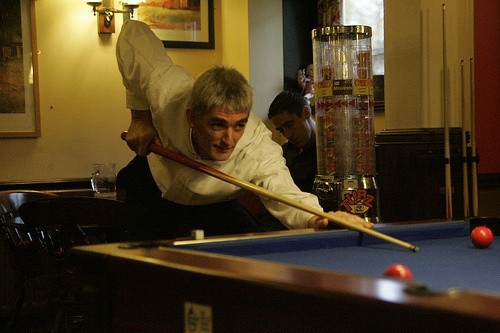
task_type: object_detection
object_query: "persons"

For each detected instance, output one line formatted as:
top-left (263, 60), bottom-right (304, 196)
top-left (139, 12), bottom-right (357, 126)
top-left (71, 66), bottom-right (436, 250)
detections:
top-left (115, 20), bottom-right (374, 242)
top-left (267, 91), bottom-right (317, 192)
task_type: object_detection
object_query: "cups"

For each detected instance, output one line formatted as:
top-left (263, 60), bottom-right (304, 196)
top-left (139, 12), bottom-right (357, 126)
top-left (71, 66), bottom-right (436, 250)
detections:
top-left (89, 162), bottom-right (117, 198)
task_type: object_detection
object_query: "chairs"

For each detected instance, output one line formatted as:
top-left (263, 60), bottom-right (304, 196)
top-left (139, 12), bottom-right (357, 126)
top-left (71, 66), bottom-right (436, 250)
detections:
top-left (0, 186), bottom-right (111, 333)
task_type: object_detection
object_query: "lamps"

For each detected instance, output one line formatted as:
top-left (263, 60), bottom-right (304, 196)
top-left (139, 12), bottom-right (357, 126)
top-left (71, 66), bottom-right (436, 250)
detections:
top-left (86, 0), bottom-right (146, 34)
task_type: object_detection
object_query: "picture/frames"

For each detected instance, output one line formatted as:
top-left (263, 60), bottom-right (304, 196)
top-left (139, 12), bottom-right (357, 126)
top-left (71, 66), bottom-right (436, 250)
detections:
top-left (123, 0), bottom-right (216, 50)
top-left (0, 0), bottom-right (42, 138)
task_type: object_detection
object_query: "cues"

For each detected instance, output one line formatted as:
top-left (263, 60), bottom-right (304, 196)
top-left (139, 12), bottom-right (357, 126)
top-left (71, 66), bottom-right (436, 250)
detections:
top-left (470, 57), bottom-right (482, 217)
top-left (460, 59), bottom-right (471, 217)
top-left (119, 130), bottom-right (421, 253)
top-left (441, 2), bottom-right (455, 220)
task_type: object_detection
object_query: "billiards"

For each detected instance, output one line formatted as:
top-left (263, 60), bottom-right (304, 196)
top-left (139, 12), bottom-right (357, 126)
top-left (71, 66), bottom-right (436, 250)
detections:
top-left (384, 264), bottom-right (414, 282)
top-left (471, 225), bottom-right (493, 248)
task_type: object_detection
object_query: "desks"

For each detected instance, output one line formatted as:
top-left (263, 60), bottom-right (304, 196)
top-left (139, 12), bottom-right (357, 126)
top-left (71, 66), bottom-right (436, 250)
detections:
top-left (65, 211), bottom-right (500, 333)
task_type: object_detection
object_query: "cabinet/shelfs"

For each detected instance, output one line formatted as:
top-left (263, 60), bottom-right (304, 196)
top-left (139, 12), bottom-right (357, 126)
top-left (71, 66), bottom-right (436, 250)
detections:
top-left (372, 127), bottom-right (474, 224)
